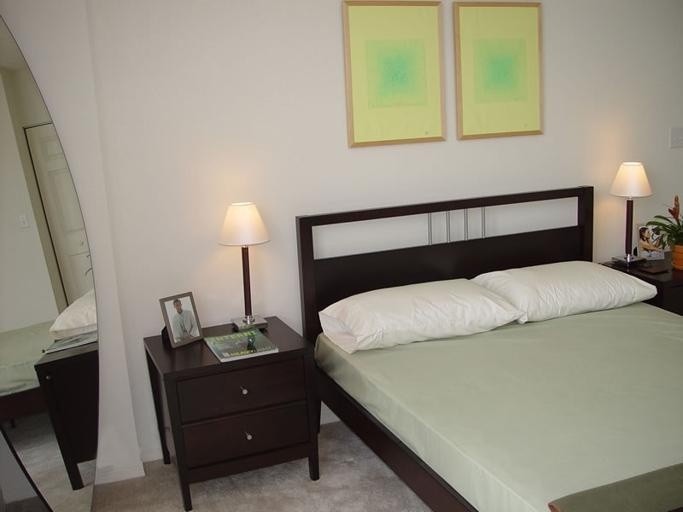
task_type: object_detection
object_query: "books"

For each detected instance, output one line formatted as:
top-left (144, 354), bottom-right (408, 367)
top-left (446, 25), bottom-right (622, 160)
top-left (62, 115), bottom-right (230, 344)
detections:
top-left (203, 329), bottom-right (279, 363)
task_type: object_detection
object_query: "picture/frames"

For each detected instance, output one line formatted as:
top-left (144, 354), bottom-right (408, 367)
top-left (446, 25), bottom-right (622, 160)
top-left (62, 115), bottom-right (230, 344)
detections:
top-left (453, 1), bottom-right (545, 141)
top-left (635, 223), bottom-right (665, 262)
top-left (159, 291), bottom-right (202, 348)
top-left (340, 0), bottom-right (447, 149)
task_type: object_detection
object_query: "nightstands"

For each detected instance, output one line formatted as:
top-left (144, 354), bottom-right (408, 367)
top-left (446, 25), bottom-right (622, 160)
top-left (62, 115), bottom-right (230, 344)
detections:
top-left (34, 331), bottom-right (99, 491)
top-left (602, 251), bottom-right (683, 316)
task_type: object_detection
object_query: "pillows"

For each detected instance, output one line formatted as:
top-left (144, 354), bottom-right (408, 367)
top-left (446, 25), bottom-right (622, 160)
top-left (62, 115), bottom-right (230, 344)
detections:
top-left (318, 279), bottom-right (526, 354)
top-left (472, 260), bottom-right (657, 324)
top-left (49, 290), bottom-right (99, 341)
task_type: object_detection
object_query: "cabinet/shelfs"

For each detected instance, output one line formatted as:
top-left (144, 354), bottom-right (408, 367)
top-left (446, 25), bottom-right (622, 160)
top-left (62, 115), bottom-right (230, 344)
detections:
top-left (143, 317), bottom-right (321, 512)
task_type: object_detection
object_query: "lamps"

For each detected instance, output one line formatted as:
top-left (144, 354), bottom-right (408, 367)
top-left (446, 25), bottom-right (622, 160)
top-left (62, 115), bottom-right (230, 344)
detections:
top-left (610, 161), bottom-right (652, 266)
top-left (217, 202), bottom-right (271, 331)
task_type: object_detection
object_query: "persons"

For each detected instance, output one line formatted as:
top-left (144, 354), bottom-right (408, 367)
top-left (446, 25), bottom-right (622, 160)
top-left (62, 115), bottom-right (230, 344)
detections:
top-left (639, 227), bottom-right (664, 257)
top-left (170, 298), bottom-right (200, 342)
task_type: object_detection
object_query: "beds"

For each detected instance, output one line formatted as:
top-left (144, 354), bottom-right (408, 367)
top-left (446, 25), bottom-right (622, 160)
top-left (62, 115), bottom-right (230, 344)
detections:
top-left (0, 287), bottom-right (96, 422)
top-left (297, 185), bottom-right (682, 512)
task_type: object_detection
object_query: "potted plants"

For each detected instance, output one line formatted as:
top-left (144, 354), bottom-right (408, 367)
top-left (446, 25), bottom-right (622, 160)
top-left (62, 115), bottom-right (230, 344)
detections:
top-left (646, 194), bottom-right (682, 269)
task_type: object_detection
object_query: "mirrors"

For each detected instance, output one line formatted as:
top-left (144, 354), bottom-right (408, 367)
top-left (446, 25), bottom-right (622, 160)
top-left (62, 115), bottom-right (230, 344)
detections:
top-left (1, 13), bottom-right (100, 512)
